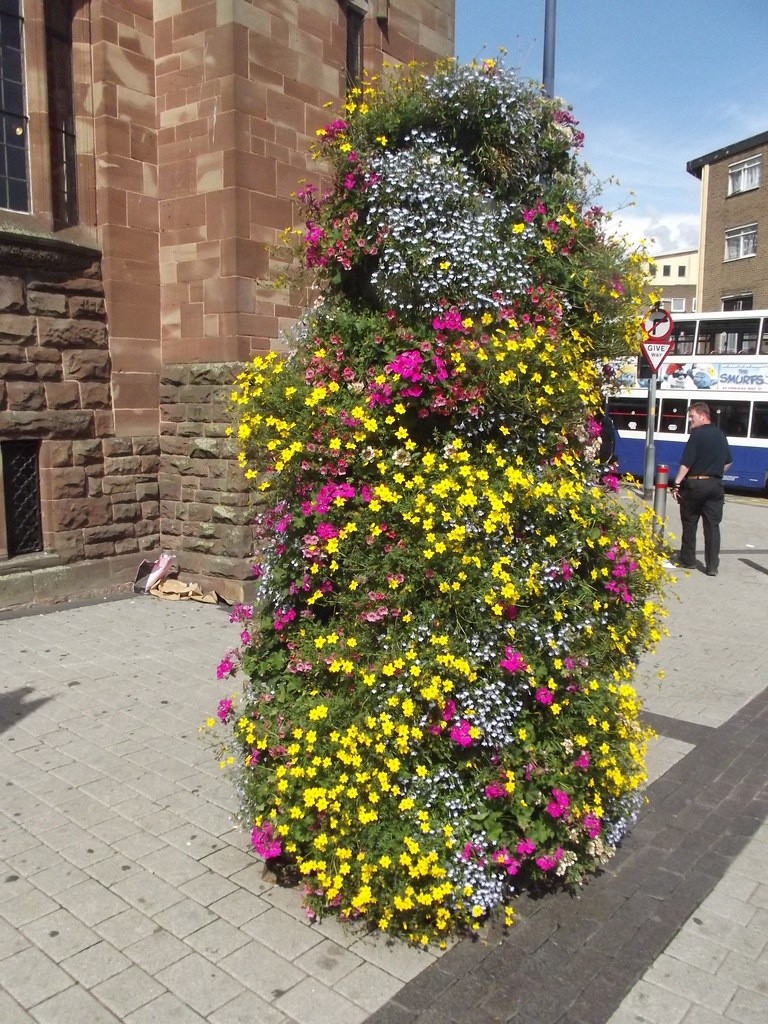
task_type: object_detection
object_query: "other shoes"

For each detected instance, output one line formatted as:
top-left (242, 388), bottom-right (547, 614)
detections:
top-left (707, 568), bottom-right (718, 575)
top-left (669, 556), bottom-right (696, 569)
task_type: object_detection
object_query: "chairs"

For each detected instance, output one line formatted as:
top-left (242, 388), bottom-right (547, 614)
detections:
top-left (683, 350), bottom-right (747, 354)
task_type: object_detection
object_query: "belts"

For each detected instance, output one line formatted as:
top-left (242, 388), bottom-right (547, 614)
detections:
top-left (684, 475), bottom-right (712, 480)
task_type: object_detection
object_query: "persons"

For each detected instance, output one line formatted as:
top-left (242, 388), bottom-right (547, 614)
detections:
top-left (670, 402), bottom-right (732, 576)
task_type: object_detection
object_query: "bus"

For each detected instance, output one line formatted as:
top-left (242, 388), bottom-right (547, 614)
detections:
top-left (586, 311), bottom-right (768, 494)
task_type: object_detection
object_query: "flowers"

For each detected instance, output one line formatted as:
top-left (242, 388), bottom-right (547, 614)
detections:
top-left (197, 49), bottom-right (678, 949)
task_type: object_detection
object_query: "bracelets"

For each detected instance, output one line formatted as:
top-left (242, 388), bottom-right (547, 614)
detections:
top-left (674, 483), bottom-right (680, 488)
